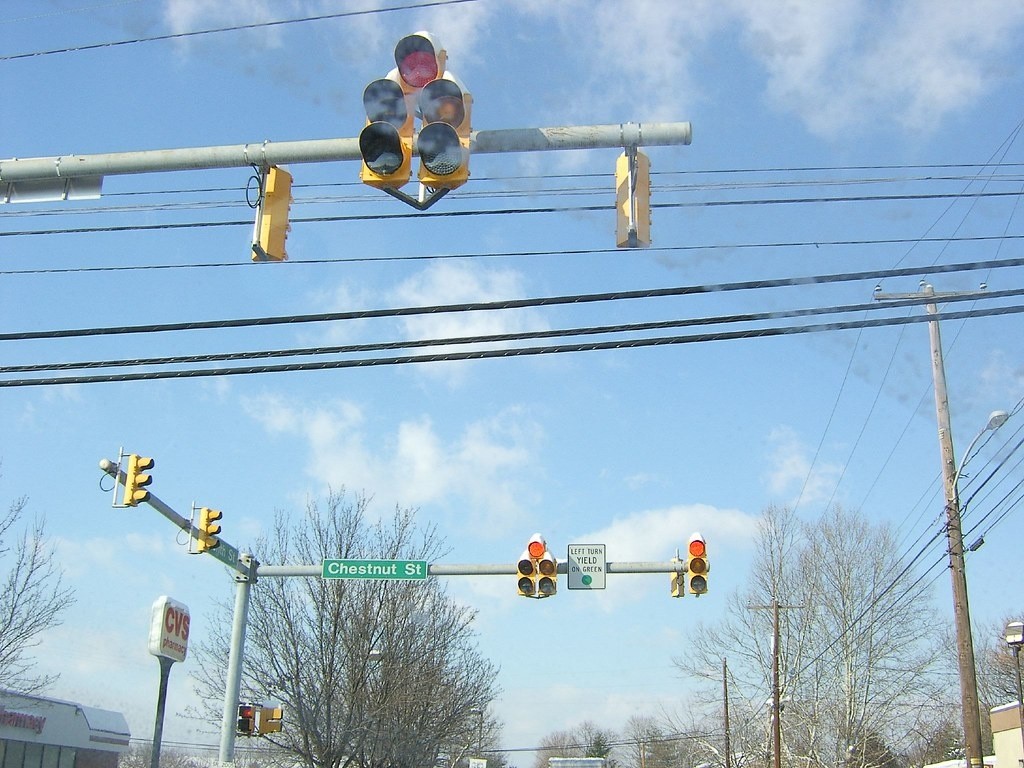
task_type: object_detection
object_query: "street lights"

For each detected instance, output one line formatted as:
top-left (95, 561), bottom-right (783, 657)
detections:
top-left (471, 708), bottom-right (480, 759)
top-left (936, 410), bottom-right (1010, 765)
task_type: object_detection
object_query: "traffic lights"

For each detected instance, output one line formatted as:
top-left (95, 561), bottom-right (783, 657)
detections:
top-left (688, 532), bottom-right (709, 597)
top-left (196, 507), bottom-right (224, 552)
top-left (238, 704), bottom-right (255, 734)
top-left (258, 707), bottom-right (285, 738)
top-left (357, 72), bottom-right (419, 191)
top-left (124, 454), bottom-right (155, 508)
top-left (412, 69), bottom-right (474, 191)
top-left (516, 550), bottom-right (537, 598)
top-left (393, 33), bottom-right (448, 89)
top-left (527, 534), bottom-right (548, 558)
top-left (537, 550), bottom-right (560, 597)
